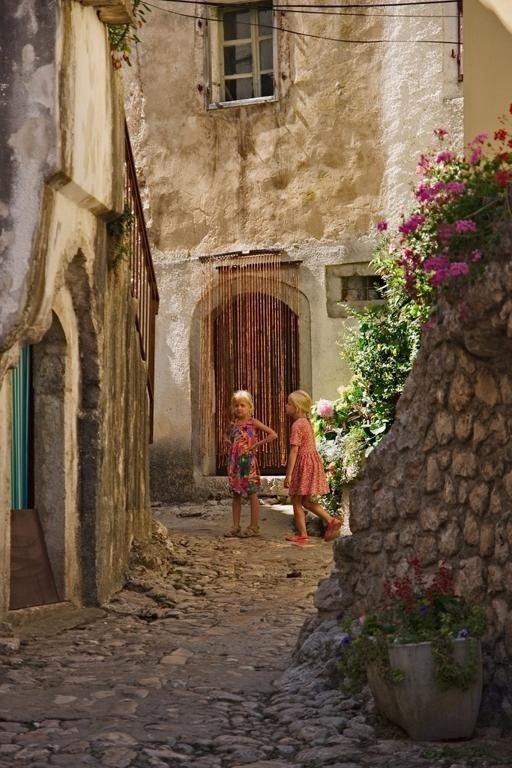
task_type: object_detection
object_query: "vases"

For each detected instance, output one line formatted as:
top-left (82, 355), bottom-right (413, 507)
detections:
top-left (366, 637), bottom-right (486, 742)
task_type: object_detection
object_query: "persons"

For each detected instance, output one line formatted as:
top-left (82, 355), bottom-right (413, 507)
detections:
top-left (282, 389), bottom-right (341, 544)
top-left (223, 390), bottom-right (278, 539)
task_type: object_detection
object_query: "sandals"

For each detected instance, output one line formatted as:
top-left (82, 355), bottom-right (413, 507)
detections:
top-left (223, 525), bottom-right (262, 538)
top-left (284, 517), bottom-right (343, 543)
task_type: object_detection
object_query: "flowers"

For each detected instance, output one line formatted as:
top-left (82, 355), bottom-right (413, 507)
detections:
top-left (333, 559), bottom-right (488, 698)
